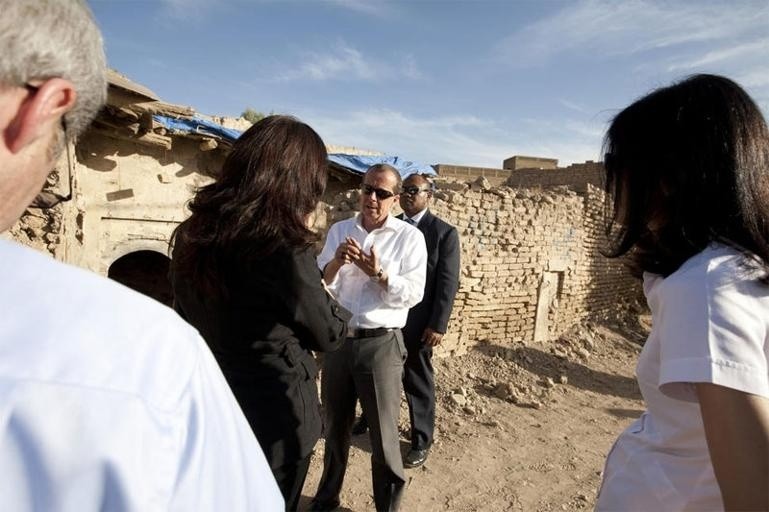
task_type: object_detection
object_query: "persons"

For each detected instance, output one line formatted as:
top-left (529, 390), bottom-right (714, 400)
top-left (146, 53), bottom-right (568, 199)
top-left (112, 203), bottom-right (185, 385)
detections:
top-left (303, 164), bottom-right (428, 512)
top-left (0, 0), bottom-right (286, 512)
top-left (352, 172), bottom-right (461, 468)
top-left (593, 74), bottom-right (769, 512)
top-left (168, 113), bottom-right (353, 512)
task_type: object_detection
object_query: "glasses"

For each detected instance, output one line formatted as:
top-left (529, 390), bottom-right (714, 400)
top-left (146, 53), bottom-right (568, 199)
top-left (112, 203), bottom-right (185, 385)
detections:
top-left (400, 187), bottom-right (429, 195)
top-left (362, 183), bottom-right (394, 200)
top-left (22, 80), bottom-right (74, 209)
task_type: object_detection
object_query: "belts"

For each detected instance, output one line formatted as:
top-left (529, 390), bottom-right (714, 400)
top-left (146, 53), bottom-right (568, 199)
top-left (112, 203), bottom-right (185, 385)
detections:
top-left (347, 327), bottom-right (394, 339)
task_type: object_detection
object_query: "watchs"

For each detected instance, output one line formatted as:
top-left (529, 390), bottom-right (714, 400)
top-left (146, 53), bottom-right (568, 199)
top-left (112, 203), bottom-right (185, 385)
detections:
top-left (370, 269), bottom-right (383, 283)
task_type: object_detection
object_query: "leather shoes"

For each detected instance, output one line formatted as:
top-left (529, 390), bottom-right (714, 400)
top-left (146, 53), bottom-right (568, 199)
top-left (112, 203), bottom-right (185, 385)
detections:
top-left (352, 417), bottom-right (368, 435)
top-left (404, 448), bottom-right (427, 469)
